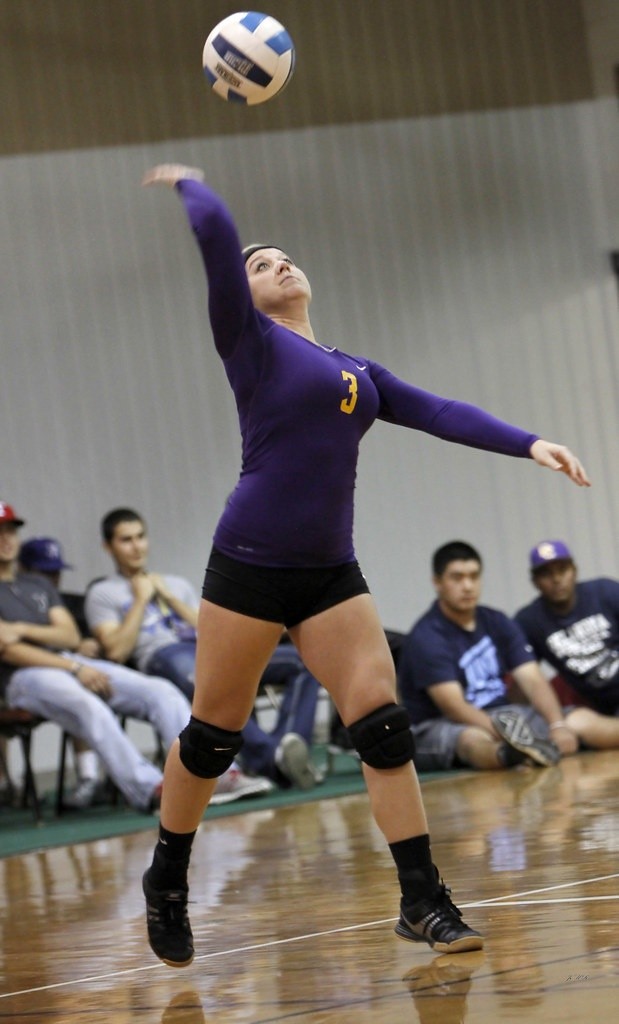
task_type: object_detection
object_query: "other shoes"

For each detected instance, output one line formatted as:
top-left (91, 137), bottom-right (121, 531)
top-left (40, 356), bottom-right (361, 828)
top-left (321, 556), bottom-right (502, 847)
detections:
top-left (492, 707), bottom-right (559, 768)
top-left (276, 732), bottom-right (320, 791)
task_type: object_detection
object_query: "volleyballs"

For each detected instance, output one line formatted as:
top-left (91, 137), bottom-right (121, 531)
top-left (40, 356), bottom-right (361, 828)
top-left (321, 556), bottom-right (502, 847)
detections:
top-left (200, 11), bottom-right (296, 107)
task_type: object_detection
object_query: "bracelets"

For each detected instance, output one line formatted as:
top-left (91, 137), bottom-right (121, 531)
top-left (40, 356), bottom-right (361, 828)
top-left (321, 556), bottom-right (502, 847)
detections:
top-left (72, 662), bottom-right (83, 675)
top-left (550, 722), bottom-right (567, 729)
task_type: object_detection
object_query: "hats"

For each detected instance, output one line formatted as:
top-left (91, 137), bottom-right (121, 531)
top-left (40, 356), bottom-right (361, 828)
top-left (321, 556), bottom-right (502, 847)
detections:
top-left (529, 541), bottom-right (571, 572)
top-left (0, 501), bottom-right (24, 529)
top-left (21, 536), bottom-right (72, 572)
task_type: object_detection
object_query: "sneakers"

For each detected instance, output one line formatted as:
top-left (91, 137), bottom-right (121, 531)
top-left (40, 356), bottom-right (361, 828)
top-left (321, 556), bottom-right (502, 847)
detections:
top-left (209, 769), bottom-right (273, 802)
top-left (143, 866), bottom-right (195, 968)
top-left (393, 878), bottom-right (484, 953)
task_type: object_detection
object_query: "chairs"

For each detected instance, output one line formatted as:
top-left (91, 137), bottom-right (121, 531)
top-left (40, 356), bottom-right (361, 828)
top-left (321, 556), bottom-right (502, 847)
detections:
top-left (0, 592), bottom-right (164, 815)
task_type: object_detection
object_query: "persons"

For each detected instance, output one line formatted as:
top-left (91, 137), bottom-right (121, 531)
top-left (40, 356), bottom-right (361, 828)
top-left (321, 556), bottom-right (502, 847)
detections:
top-left (398, 540), bottom-right (576, 770)
top-left (516, 541), bottom-right (619, 750)
top-left (141, 164), bottom-right (590, 967)
top-left (87, 508), bottom-right (321, 789)
top-left (1, 502), bottom-right (272, 817)
